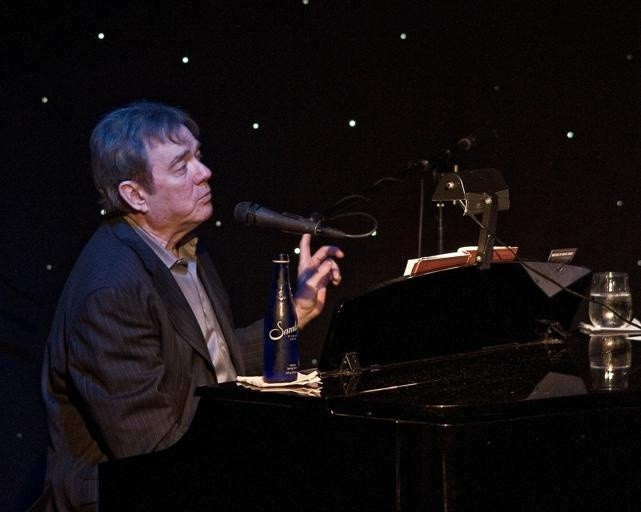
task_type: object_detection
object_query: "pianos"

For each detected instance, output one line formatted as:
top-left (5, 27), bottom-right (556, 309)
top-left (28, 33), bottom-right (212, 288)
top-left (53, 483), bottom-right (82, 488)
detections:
top-left (99, 260), bottom-right (641, 511)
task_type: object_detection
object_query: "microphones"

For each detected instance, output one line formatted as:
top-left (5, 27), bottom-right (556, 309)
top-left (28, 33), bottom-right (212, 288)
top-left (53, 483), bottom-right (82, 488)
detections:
top-left (442, 135), bottom-right (478, 160)
top-left (394, 158), bottom-right (433, 175)
top-left (234, 200), bottom-right (346, 238)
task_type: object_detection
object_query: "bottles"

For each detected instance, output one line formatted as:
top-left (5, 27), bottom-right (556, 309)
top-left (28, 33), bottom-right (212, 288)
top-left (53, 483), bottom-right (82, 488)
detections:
top-left (260, 253), bottom-right (299, 384)
top-left (587, 271), bottom-right (633, 330)
top-left (585, 333), bottom-right (632, 392)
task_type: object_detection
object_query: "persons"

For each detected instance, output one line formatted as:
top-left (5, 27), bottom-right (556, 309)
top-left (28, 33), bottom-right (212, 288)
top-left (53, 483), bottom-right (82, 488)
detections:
top-left (42, 101), bottom-right (345, 511)
